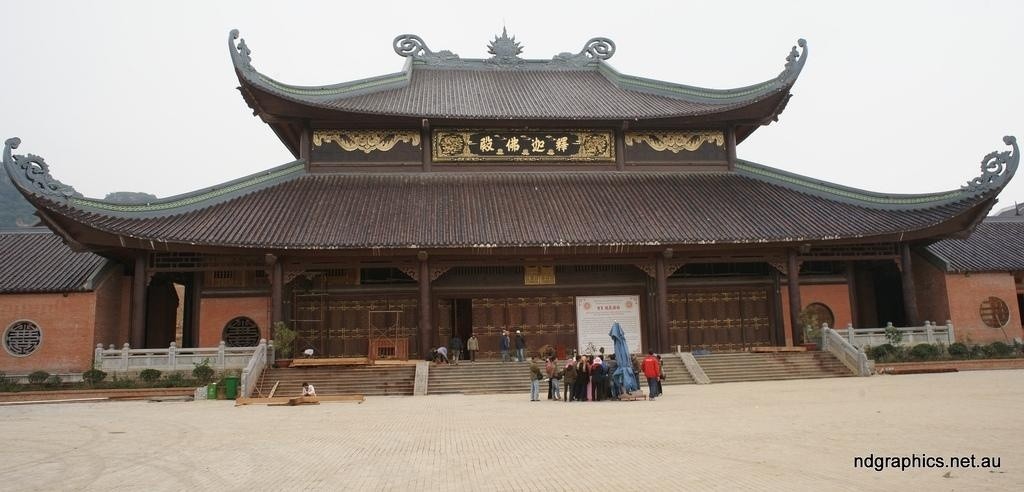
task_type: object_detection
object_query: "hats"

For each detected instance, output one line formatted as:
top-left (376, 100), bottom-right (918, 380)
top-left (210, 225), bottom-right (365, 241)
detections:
top-left (592, 357), bottom-right (602, 364)
top-left (563, 359), bottom-right (575, 368)
top-left (515, 330), bottom-right (520, 334)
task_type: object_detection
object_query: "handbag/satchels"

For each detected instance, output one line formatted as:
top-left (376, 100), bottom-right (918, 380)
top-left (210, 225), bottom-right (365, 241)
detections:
top-left (536, 369), bottom-right (543, 380)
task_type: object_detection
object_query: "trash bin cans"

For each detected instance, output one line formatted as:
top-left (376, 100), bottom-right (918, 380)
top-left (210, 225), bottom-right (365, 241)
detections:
top-left (224, 376), bottom-right (240, 399)
top-left (208, 382), bottom-right (217, 399)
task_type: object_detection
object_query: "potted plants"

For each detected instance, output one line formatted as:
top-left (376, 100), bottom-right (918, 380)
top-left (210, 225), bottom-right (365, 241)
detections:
top-left (797, 310), bottom-right (820, 350)
top-left (271, 320), bottom-right (296, 368)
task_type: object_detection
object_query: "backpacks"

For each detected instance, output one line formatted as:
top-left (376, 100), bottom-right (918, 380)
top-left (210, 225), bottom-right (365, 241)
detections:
top-left (660, 367), bottom-right (665, 379)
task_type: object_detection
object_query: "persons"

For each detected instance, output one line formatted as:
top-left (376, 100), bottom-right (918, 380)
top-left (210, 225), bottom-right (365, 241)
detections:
top-left (529, 348), bottom-right (665, 401)
top-left (300, 382), bottom-right (315, 396)
top-left (425, 329), bottom-right (527, 364)
